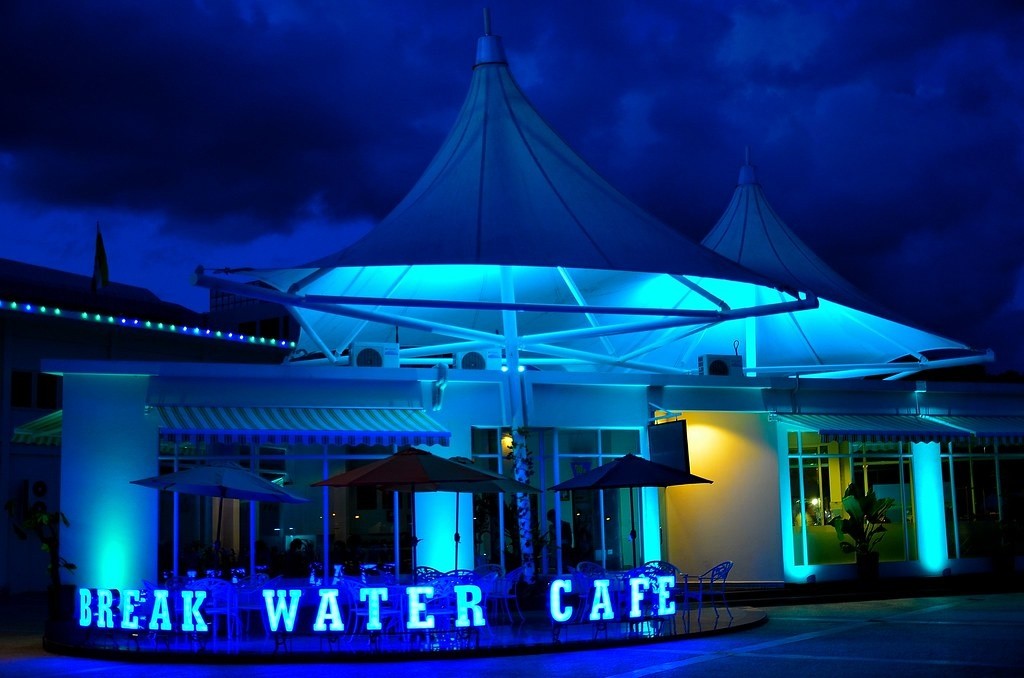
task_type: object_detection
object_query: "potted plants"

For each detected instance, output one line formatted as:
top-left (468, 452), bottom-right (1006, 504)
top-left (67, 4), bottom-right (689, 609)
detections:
top-left (828, 484), bottom-right (895, 562)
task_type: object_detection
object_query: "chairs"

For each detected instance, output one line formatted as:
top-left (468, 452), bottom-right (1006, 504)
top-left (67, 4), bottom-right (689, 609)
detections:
top-left (566, 560), bottom-right (733, 622)
top-left (334, 566), bottom-right (526, 629)
top-left (141, 575), bottom-right (281, 638)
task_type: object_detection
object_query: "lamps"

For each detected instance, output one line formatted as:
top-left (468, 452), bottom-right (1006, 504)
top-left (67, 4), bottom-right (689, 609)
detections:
top-left (912, 380), bottom-right (927, 393)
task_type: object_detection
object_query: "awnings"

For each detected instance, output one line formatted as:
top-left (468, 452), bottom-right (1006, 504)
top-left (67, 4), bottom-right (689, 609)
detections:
top-left (144, 403), bottom-right (451, 448)
top-left (918, 415), bottom-right (1024, 445)
top-left (783, 412), bottom-right (971, 447)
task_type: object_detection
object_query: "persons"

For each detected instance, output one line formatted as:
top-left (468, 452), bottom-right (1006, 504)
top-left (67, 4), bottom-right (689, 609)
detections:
top-left (576, 509), bottom-right (595, 562)
top-left (795, 501), bottom-right (816, 526)
top-left (158, 534), bottom-right (319, 574)
top-left (547, 513), bottom-right (571, 559)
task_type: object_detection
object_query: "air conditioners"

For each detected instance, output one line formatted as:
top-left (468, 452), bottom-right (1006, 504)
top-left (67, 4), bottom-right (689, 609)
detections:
top-left (452, 348), bottom-right (503, 370)
top-left (347, 341), bottom-right (400, 368)
top-left (697, 354), bottom-right (743, 376)
top-left (25, 478), bottom-right (50, 503)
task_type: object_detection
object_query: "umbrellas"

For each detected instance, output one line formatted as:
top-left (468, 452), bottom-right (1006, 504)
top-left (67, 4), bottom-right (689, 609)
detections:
top-left (131, 467), bottom-right (312, 568)
top-left (311, 446), bottom-right (504, 584)
top-left (549, 452), bottom-right (713, 568)
top-left (375, 455), bottom-right (546, 577)
